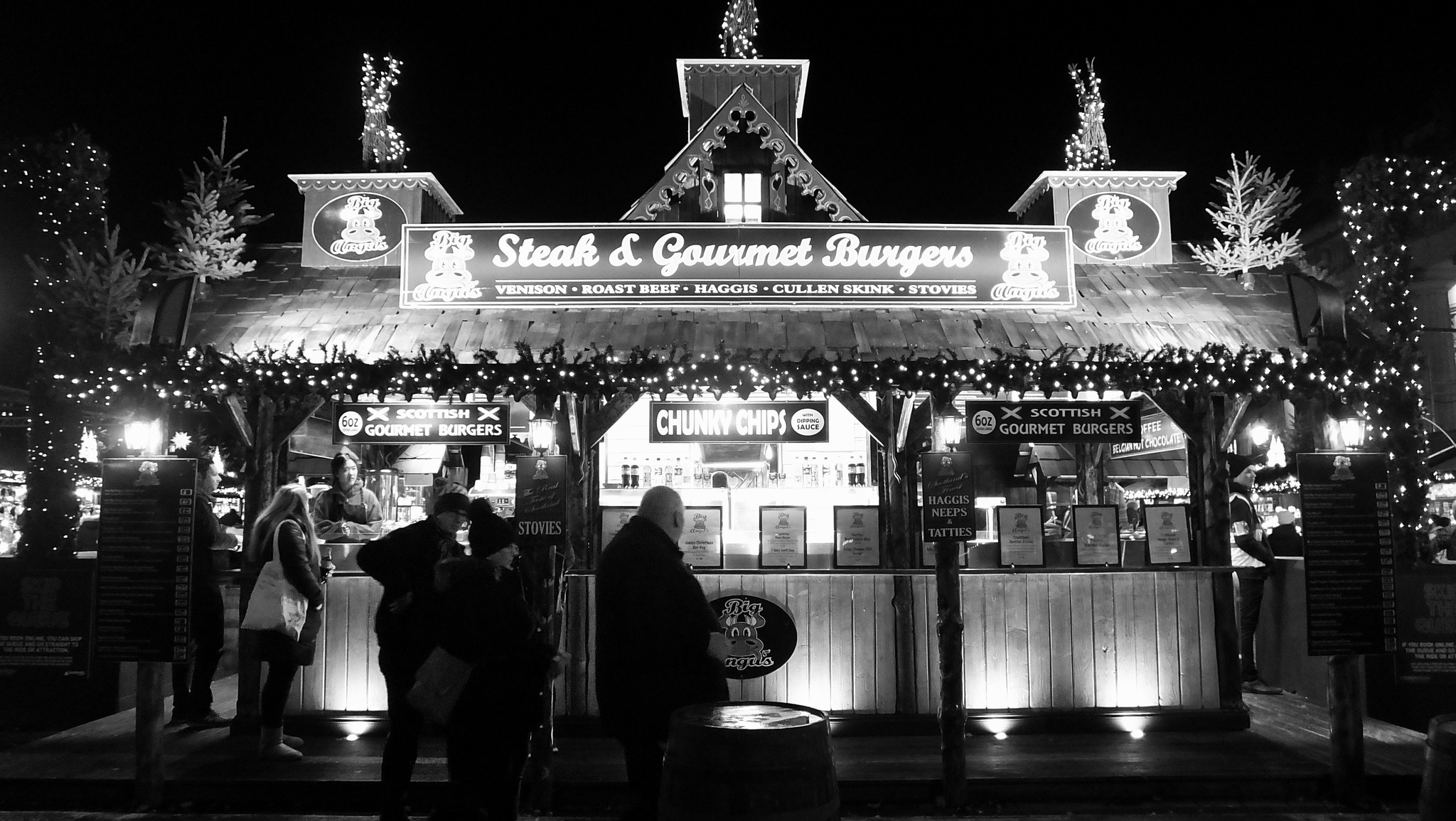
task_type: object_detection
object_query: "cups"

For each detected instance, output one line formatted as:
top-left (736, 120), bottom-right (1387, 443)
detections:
top-left (474, 445), bottom-right (506, 492)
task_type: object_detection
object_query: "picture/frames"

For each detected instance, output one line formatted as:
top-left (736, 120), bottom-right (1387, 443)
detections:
top-left (1070, 503), bottom-right (1122, 567)
top-left (674, 505), bottom-right (724, 570)
top-left (832, 505), bottom-right (883, 569)
top-left (995, 504), bottom-right (1047, 569)
top-left (758, 505), bottom-right (808, 570)
top-left (598, 505), bottom-right (640, 555)
top-left (919, 506), bottom-right (969, 569)
top-left (1140, 502), bottom-right (1193, 567)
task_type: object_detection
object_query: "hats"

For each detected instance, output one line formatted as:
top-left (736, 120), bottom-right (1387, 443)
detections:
top-left (433, 483), bottom-right (470, 516)
top-left (1227, 450), bottom-right (1254, 479)
top-left (466, 498), bottom-right (516, 560)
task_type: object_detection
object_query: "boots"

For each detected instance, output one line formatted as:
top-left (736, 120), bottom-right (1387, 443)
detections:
top-left (282, 732), bottom-right (304, 749)
top-left (259, 723), bottom-right (303, 762)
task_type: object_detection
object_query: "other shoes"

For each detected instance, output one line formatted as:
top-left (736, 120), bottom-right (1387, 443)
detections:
top-left (193, 710), bottom-right (234, 730)
top-left (171, 709), bottom-right (187, 724)
top-left (1241, 679), bottom-right (1283, 694)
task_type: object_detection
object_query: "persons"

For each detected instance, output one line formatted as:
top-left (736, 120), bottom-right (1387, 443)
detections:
top-left (596, 485), bottom-right (710, 821)
top-left (1127, 454), bottom-right (1456, 697)
top-left (169, 447), bottom-right (578, 821)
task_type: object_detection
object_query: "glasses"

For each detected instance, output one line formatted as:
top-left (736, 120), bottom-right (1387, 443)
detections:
top-left (446, 508), bottom-right (467, 520)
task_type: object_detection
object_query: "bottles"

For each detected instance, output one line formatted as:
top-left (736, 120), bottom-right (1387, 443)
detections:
top-left (620, 457), bottom-right (713, 490)
top-left (793, 454), bottom-right (866, 487)
top-left (320, 546), bottom-right (332, 585)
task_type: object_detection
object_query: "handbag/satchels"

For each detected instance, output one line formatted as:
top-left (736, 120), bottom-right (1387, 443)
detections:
top-left (404, 646), bottom-right (471, 725)
top-left (240, 521), bottom-right (310, 642)
